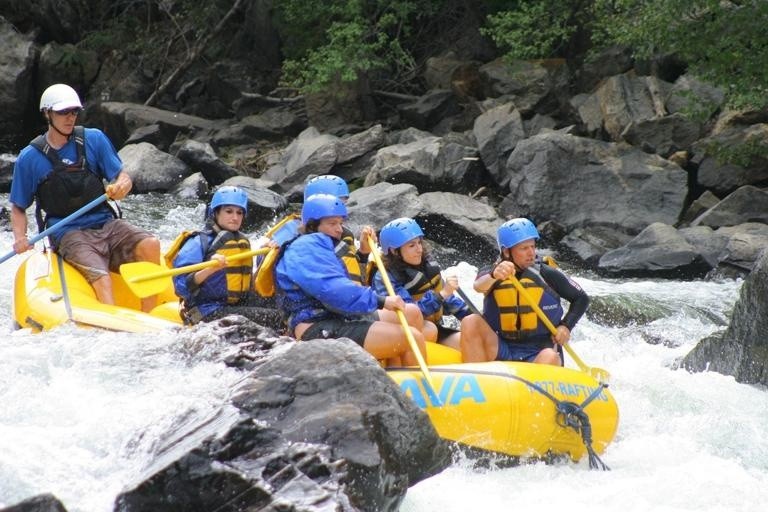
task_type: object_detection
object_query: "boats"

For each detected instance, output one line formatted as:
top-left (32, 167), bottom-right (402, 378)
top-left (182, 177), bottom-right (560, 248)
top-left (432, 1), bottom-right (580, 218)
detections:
top-left (14, 251), bottom-right (620, 464)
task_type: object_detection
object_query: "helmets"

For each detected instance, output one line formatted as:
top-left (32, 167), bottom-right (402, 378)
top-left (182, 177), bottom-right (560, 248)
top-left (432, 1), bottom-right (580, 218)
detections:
top-left (39, 84), bottom-right (84, 114)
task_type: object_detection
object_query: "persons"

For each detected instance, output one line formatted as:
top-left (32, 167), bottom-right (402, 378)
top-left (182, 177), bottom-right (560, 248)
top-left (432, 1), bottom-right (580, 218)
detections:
top-left (272, 195), bottom-right (429, 368)
top-left (9, 82), bottom-right (161, 313)
top-left (170, 187), bottom-right (285, 336)
top-left (287, 173), bottom-right (368, 342)
top-left (371, 217), bottom-right (474, 354)
top-left (461, 217), bottom-right (589, 367)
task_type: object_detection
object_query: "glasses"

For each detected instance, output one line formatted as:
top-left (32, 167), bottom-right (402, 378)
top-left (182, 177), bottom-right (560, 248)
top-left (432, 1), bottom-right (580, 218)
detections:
top-left (57, 109), bottom-right (79, 115)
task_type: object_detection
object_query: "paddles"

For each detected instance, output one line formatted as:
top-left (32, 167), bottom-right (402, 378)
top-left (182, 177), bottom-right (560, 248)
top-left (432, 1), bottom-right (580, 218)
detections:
top-left (120, 250), bottom-right (265, 298)
